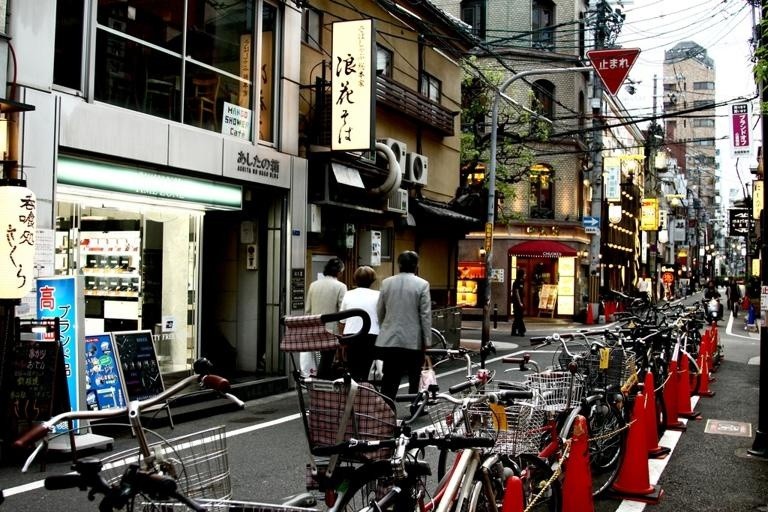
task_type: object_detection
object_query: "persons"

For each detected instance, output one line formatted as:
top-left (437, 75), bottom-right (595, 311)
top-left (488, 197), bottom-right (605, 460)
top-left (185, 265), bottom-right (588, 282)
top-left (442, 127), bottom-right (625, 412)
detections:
top-left (375, 249), bottom-right (433, 418)
top-left (302, 257), bottom-right (351, 380)
top-left (510, 269), bottom-right (528, 337)
top-left (702, 275), bottom-right (742, 320)
top-left (336, 265), bottom-right (384, 382)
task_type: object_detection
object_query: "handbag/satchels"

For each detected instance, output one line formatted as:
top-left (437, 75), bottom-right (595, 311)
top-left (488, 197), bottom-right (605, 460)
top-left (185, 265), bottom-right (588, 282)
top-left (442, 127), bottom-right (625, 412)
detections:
top-left (418, 369), bottom-right (438, 396)
top-left (368, 360), bottom-right (384, 381)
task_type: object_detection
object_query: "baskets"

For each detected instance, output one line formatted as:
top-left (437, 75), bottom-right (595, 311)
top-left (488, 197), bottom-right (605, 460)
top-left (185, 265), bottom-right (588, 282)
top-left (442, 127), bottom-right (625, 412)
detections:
top-left (98, 425), bottom-right (231, 512)
top-left (586, 349), bottom-right (636, 388)
top-left (433, 362), bottom-right (576, 459)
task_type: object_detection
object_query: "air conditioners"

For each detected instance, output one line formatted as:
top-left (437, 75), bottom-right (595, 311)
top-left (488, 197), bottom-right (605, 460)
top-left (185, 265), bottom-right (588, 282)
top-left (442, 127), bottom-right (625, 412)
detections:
top-left (402, 152), bottom-right (428, 185)
top-left (376, 137), bottom-right (407, 174)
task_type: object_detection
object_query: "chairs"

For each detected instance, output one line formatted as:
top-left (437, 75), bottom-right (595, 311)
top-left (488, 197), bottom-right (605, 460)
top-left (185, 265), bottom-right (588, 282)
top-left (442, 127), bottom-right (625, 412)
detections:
top-left (110, 60), bottom-right (222, 132)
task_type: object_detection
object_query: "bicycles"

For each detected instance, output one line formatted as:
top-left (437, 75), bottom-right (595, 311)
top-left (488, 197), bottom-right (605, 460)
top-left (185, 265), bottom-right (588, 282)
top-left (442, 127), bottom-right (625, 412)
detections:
top-left (1, 355), bottom-right (249, 512)
top-left (270, 295), bottom-right (704, 512)
top-left (39, 453), bottom-right (204, 511)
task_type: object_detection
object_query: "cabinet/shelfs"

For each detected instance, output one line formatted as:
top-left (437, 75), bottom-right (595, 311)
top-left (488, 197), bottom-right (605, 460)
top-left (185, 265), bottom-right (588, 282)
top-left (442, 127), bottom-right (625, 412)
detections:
top-left (58, 219), bottom-right (162, 335)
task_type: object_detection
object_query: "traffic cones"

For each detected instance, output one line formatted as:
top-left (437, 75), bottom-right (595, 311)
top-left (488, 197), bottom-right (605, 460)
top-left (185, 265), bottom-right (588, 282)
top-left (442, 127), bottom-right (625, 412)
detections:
top-left (641, 372), bottom-right (671, 459)
top-left (676, 354), bottom-right (702, 420)
top-left (659, 361), bottom-right (687, 432)
top-left (596, 393), bottom-right (664, 505)
top-left (502, 476), bottom-right (524, 512)
top-left (583, 304), bottom-right (594, 325)
top-left (561, 414), bottom-right (595, 512)
top-left (699, 321), bottom-right (724, 399)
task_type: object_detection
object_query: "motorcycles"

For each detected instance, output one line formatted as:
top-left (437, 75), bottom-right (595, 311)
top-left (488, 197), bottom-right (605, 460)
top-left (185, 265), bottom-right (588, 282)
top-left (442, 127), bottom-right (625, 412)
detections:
top-left (703, 297), bottom-right (724, 326)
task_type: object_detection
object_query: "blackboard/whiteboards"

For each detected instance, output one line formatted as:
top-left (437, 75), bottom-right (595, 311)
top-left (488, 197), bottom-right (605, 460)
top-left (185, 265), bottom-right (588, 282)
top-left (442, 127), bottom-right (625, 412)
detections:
top-left (0, 339), bottom-right (61, 452)
top-left (111, 329), bottom-right (169, 413)
